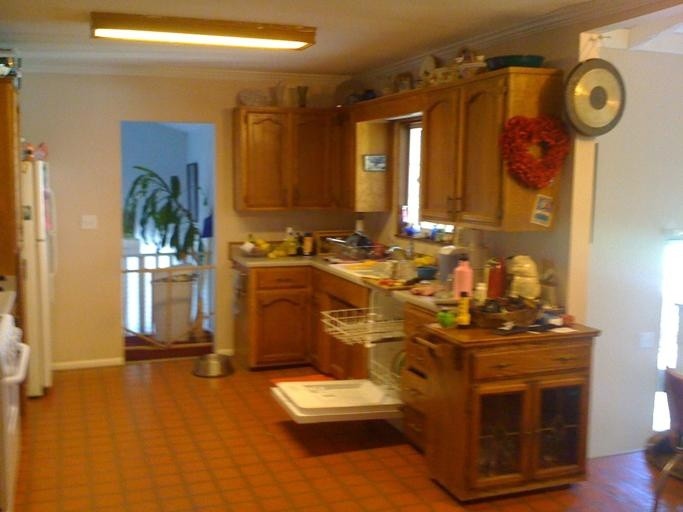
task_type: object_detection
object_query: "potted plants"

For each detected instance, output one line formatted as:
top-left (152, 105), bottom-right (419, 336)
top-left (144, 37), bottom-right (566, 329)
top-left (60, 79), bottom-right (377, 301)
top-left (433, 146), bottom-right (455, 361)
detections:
top-left (122, 166), bottom-right (213, 343)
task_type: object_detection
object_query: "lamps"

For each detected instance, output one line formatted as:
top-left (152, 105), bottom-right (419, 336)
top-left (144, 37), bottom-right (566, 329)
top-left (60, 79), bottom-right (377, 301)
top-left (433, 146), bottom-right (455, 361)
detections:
top-left (89, 11), bottom-right (317, 51)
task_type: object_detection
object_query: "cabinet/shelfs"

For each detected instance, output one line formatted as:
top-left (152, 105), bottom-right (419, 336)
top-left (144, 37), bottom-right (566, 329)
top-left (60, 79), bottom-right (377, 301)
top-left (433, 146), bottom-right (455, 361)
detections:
top-left (422, 72), bottom-right (563, 233)
top-left (232, 107), bottom-right (288, 209)
top-left (333, 108), bottom-right (392, 211)
top-left (314, 267), bottom-right (368, 381)
top-left (0, 77), bottom-right (26, 411)
top-left (293, 108), bottom-right (331, 209)
top-left (400, 302), bottom-right (444, 455)
top-left (428, 322), bottom-right (602, 501)
top-left (233, 266), bottom-right (313, 366)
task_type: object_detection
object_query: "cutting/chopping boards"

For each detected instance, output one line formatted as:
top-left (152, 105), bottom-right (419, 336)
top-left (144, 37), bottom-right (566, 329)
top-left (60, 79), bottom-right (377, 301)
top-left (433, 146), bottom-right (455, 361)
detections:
top-left (361, 278), bottom-right (430, 291)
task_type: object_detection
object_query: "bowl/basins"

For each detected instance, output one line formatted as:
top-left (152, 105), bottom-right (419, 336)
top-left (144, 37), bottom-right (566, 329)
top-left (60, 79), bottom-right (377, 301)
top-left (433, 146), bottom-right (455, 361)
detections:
top-left (473, 295), bottom-right (541, 334)
top-left (269, 85), bottom-right (309, 107)
top-left (239, 247), bottom-right (272, 260)
top-left (191, 353), bottom-right (233, 378)
top-left (416, 266), bottom-right (436, 277)
top-left (430, 55), bottom-right (542, 85)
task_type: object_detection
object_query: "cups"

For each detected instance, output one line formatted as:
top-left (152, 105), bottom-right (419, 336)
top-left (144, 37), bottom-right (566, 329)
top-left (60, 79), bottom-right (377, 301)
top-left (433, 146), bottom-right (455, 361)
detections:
top-left (375, 244), bottom-right (386, 258)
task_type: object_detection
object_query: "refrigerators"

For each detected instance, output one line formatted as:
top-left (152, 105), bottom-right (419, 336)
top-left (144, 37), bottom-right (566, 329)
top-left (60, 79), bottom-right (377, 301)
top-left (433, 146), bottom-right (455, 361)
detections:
top-left (16, 161), bottom-right (55, 398)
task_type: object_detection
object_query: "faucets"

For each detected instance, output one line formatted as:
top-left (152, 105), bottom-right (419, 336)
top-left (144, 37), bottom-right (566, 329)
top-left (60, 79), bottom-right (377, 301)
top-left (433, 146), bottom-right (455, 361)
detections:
top-left (384, 238), bottom-right (415, 260)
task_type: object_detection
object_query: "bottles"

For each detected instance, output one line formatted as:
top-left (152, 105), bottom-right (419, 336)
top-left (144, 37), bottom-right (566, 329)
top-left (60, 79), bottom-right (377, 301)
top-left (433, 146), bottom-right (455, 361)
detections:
top-left (439, 251), bottom-right (504, 327)
top-left (284, 226), bottom-right (314, 256)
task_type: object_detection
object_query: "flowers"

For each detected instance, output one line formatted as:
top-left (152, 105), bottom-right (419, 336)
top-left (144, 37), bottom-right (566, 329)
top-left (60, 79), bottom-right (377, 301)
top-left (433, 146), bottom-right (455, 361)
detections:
top-left (501, 115), bottom-right (570, 191)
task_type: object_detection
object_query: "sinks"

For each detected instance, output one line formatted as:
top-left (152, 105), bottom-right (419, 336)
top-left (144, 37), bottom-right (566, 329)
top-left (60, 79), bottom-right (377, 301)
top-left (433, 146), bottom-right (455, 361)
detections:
top-left (348, 272), bottom-right (393, 288)
top-left (328, 262), bottom-right (388, 271)
